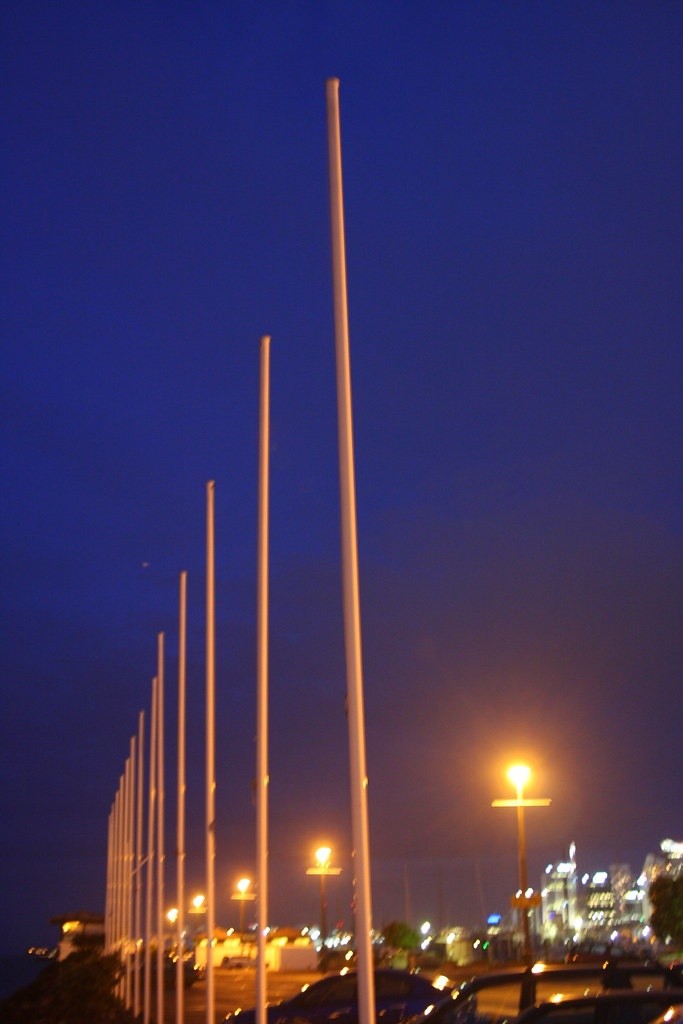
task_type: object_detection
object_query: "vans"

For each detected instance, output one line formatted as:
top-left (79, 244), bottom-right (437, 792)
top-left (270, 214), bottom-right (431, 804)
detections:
top-left (404, 964), bottom-right (683, 1024)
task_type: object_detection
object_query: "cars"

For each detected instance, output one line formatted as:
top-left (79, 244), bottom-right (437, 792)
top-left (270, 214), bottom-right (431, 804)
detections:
top-left (132, 957), bottom-right (205, 989)
top-left (222, 967), bottom-right (477, 1024)
top-left (507, 989), bottom-right (683, 1024)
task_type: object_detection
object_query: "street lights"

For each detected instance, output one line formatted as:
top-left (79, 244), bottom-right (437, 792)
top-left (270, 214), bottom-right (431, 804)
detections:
top-left (186, 895), bottom-right (209, 932)
top-left (304, 846), bottom-right (343, 974)
top-left (492, 763), bottom-right (554, 966)
top-left (230, 876), bottom-right (257, 930)
